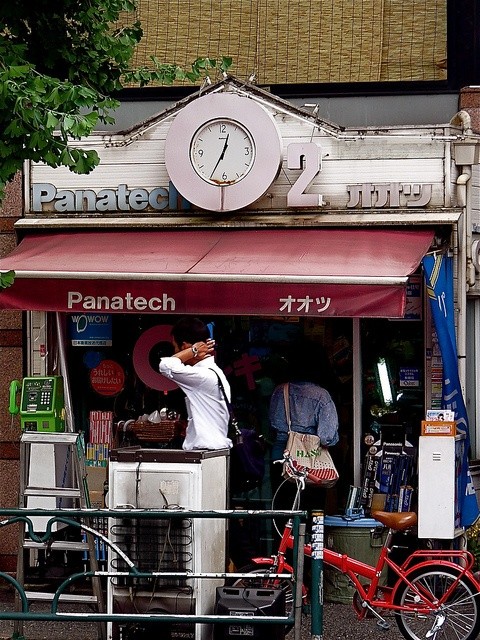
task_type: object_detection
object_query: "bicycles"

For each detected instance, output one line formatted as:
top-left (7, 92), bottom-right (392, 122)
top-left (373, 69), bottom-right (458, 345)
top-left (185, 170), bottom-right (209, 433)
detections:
top-left (223, 449), bottom-right (480, 640)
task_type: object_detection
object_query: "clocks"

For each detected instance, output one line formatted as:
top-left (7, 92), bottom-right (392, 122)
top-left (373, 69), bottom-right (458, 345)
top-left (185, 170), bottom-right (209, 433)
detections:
top-left (190, 118), bottom-right (257, 188)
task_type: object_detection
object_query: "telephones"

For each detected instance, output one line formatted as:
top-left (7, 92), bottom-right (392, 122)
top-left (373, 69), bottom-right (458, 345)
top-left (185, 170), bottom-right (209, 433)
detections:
top-left (8, 376), bottom-right (64, 433)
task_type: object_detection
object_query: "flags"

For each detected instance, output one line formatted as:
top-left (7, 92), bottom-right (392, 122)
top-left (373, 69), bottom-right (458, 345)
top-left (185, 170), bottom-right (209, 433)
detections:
top-left (418, 246), bottom-right (480, 532)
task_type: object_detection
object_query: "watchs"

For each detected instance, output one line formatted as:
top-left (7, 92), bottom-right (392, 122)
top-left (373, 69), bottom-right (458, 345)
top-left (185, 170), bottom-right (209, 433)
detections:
top-left (191, 345), bottom-right (198, 358)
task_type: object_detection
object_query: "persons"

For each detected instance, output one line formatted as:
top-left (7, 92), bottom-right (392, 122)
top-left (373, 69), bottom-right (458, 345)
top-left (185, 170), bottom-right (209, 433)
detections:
top-left (155, 317), bottom-right (233, 451)
top-left (264, 349), bottom-right (340, 555)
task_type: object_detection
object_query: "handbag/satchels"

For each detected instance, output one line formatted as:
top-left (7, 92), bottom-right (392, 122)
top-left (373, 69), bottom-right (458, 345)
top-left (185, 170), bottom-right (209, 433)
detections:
top-left (229, 423), bottom-right (267, 493)
top-left (280, 432), bottom-right (340, 488)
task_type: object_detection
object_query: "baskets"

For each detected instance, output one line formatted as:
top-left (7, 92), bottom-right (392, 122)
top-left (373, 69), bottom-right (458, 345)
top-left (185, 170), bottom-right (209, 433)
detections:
top-left (116, 419), bottom-right (179, 444)
